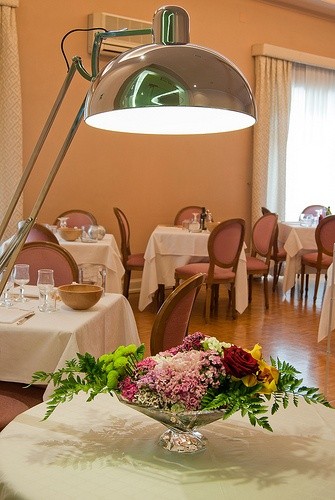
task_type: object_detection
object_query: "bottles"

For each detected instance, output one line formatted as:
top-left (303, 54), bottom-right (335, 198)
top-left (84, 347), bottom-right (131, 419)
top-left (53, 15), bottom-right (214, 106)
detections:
top-left (319, 214), bottom-right (323, 222)
top-left (88, 225), bottom-right (106, 240)
top-left (200, 207), bottom-right (212, 231)
top-left (326, 206), bottom-right (332, 216)
top-left (189, 212), bottom-right (200, 233)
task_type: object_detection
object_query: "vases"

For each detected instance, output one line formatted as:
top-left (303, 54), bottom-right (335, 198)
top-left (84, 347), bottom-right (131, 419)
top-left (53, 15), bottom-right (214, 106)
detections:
top-left (114, 391), bottom-right (228, 454)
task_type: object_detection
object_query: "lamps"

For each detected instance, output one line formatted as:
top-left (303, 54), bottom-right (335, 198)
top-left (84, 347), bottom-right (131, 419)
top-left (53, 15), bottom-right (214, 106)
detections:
top-left (0, 5), bottom-right (256, 295)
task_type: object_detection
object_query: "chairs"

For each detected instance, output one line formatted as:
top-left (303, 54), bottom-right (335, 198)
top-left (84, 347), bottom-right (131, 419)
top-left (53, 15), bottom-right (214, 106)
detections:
top-left (0, 203), bottom-right (335, 430)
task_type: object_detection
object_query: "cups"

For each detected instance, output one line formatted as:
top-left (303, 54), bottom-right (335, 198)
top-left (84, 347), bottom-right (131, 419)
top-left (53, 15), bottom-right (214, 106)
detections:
top-left (299, 209), bottom-right (322, 227)
top-left (48, 226), bottom-right (57, 234)
top-left (182, 218), bottom-right (192, 232)
top-left (80, 225), bottom-right (98, 243)
top-left (41, 224), bottom-right (48, 228)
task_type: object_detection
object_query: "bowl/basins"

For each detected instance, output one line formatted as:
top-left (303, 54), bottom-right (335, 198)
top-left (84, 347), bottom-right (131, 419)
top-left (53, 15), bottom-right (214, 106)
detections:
top-left (206, 222), bottom-right (220, 234)
top-left (57, 228), bottom-right (81, 241)
top-left (58, 284), bottom-right (104, 310)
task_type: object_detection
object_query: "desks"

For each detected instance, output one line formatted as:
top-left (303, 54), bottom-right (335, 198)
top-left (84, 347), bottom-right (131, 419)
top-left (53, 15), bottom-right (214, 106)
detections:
top-left (0, 220), bottom-right (335, 500)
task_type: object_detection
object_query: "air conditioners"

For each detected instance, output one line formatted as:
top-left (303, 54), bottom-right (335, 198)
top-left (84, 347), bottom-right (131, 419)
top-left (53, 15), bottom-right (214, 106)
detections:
top-left (87, 13), bottom-right (154, 58)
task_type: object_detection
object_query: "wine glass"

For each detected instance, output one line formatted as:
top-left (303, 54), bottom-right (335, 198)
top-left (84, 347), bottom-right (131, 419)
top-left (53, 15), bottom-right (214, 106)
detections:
top-left (37, 268), bottom-right (54, 311)
top-left (13, 264), bottom-right (31, 302)
top-left (57, 217), bottom-right (70, 228)
top-left (0, 268), bottom-right (14, 306)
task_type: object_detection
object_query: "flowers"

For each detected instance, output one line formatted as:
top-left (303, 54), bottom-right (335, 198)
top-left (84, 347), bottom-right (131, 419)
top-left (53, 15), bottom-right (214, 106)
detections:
top-left (21, 331), bottom-right (335, 432)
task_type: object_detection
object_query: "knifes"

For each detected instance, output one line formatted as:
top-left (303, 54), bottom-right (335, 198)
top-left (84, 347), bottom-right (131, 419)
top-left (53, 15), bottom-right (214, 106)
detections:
top-left (17, 314), bottom-right (34, 325)
